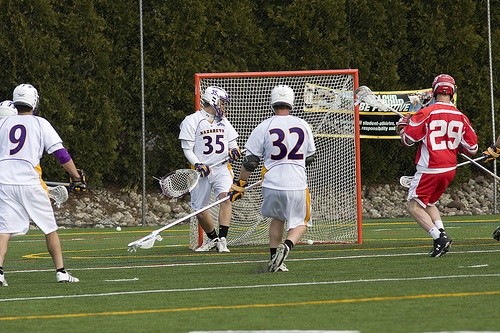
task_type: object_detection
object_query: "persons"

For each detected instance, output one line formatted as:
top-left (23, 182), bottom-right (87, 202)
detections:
top-left (0, 83), bottom-right (86, 287)
top-left (396, 75), bottom-right (500, 257)
top-left (178, 86), bottom-right (244, 252)
top-left (226, 85), bottom-right (316, 273)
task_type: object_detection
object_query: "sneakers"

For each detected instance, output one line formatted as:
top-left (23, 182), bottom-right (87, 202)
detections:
top-left (55, 268), bottom-right (79, 283)
top-left (429, 228), bottom-right (452, 258)
top-left (196, 235), bottom-right (230, 253)
top-left (267, 243), bottom-right (290, 272)
top-left (0, 273), bottom-right (8, 286)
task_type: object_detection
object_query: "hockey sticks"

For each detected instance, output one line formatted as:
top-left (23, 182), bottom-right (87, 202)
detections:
top-left (44, 181), bottom-right (71, 186)
top-left (49, 185), bottom-right (71, 206)
top-left (160, 147), bottom-right (245, 199)
top-left (354, 86), bottom-right (500, 180)
top-left (400, 154), bottom-right (492, 188)
top-left (127, 179), bottom-right (265, 249)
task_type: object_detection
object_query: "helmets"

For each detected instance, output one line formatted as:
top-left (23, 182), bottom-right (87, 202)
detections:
top-left (271, 85), bottom-right (295, 107)
top-left (12, 83), bottom-right (38, 110)
top-left (432, 74), bottom-right (455, 98)
top-left (0, 100), bottom-right (18, 118)
top-left (202, 86), bottom-right (228, 123)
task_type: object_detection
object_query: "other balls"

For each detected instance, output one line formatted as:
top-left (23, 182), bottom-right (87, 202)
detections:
top-left (116, 227), bottom-right (121, 231)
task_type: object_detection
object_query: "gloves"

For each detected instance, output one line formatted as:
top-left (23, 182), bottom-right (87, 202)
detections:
top-left (226, 177), bottom-right (249, 203)
top-left (229, 148), bottom-right (242, 163)
top-left (68, 169), bottom-right (86, 194)
top-left (478, 144), bottom-right (500, 164)
top-left (195, 163), bottom-right (210, 178)
top-left (397, 112), bottom-right (409, 133)
